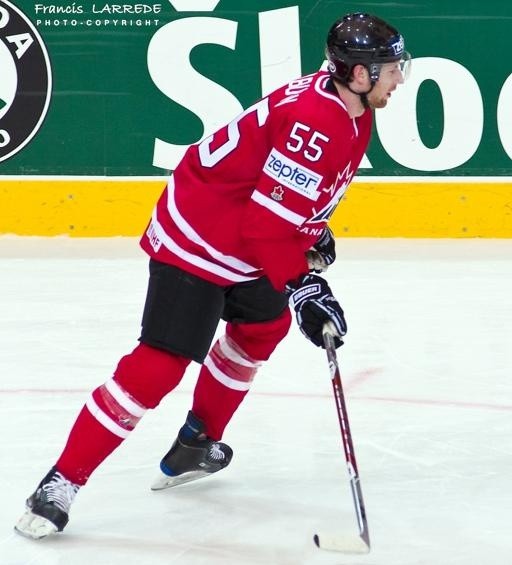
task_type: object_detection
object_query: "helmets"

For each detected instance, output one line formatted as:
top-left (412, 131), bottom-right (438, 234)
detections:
top-left (324, 11), bottom-right (411, 84)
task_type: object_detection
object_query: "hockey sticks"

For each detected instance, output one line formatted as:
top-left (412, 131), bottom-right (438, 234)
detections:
top-left (314, 325), bottom-right (374, 556)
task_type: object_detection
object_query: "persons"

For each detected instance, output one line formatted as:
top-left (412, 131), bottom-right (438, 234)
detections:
top-left (14, 11), bottom-right (411, 542)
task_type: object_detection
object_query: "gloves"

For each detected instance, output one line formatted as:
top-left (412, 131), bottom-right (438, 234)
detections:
top-left (308, 227), bottom-right (336, 274)
top-left (284, 273), bottom-right (347, 349)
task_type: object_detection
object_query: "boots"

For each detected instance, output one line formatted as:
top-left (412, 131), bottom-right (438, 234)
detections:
top-left (25, 467), bottom-right (80, 531)
top-left (160, 411), bottom-right (233, 477)
